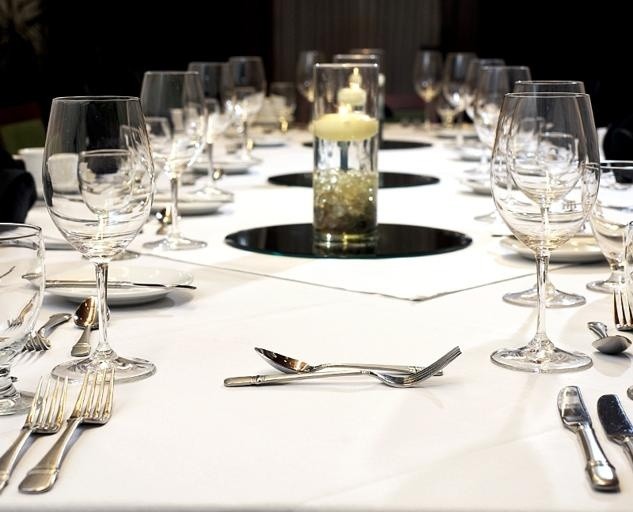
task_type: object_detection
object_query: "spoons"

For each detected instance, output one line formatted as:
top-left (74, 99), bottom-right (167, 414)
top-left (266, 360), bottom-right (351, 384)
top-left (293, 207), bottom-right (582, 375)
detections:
top-left (254, 346), bottom-right (444, 377)
top-left (72, 295), bottom-right (110, 356)
top-left (588, 320), bottom-right (632, 352)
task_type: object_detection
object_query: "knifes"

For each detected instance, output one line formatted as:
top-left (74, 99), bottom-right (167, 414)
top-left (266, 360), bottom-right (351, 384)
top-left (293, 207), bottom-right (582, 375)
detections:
top-left (557, 386), bottom-right (620, 491)
top-left (596, 394), bottom-right (633, 464)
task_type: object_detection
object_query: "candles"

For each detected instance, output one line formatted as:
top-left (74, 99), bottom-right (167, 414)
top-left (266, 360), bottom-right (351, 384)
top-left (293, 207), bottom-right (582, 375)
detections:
top-left (313, 102), bottom-right (376, 141)
top-left (337, 84), bottom-right (367, 106)
top-left (349, 68), bottom-right (362, 84)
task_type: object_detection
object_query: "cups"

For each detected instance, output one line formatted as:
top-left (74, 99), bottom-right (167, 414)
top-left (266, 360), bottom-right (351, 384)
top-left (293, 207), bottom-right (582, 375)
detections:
top-left (140, 71), bottom-right (209, 251)
top-left (41, 95), bottom-right (157, 385)
top-left (221, 58), bottom-right (266, 162)
top-left (441, 52), bottom-right (476, 154)
top-left (413, 48), bottom-right (448, 132)
top-left (464, 57), bottom-right (507, 160)
top-left (298, 49), bottom-right (323, 105)
top-left (512, 78), bottom-right (592, 250)
top-left (184, 61), bottom-right (238, 172)
top-left (0, 224), bottom-right (46, 417)
top-left (311, 64), bottom-right (383, 253)
top-left (489, 92), bottom-right (603, 375)
top-left (479, 65), bottom-right (536, 209)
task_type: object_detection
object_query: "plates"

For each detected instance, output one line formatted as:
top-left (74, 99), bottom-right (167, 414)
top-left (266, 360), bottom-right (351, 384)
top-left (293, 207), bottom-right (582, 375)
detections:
top-left (502, 236), bottom-right (605, 261)
top-left (463, 178), bottom-right (494, 194)
top-left (189, 159), bottom-right (253, 173)
top-left (437, 129), bottom-right (475, 139)
top-left (31, 262), bottom-right (194, 307)
top-left (149, 201), bottom-right (221, 215)
top-left (250, 138), bottom-right (288, 147)
top-left (461, 150), bottom-right (505, 163)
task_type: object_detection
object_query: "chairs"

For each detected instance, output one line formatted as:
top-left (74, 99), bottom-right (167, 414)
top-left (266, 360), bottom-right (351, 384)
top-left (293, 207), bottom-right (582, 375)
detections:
top-left (1, 121), bottom-right (45, 153)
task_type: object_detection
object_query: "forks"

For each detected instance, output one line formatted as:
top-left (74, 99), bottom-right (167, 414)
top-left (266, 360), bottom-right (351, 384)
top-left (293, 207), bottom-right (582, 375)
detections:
top-left (23, 313), bottom-right (72, 352)
top-left (17, 368), bottom-right (117, 494)
top-left (613, 290), bottom-right (632, 331)
top-left (224, 346), bottom-right (463, 389)
top-left (0, 375), bottom-right (69, 494)
